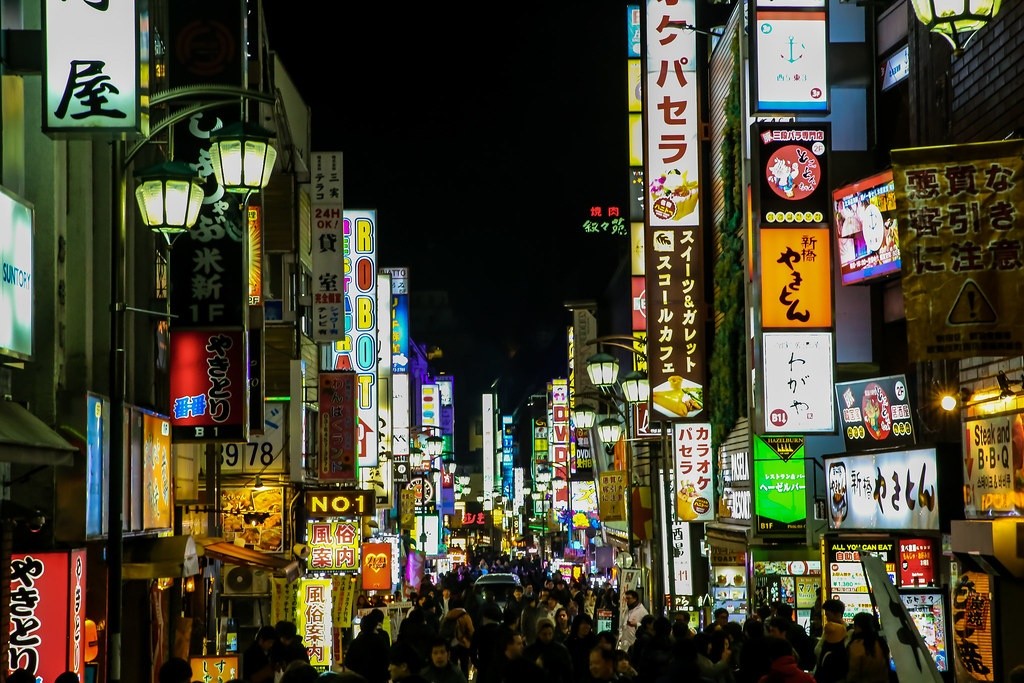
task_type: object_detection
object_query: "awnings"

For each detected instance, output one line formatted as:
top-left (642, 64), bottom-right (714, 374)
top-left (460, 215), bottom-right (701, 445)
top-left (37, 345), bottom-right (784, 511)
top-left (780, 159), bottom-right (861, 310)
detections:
top-left (122, 534), bottom-right (199, 581)
top-left (204, 542), bottom-right (299, 584)
top-left (0, 401), bottom-right (79, 467)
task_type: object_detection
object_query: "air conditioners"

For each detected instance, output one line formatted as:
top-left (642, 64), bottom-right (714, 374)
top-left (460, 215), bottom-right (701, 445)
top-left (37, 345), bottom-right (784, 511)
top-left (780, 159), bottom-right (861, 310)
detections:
top-left (223, 563), bottom-right (268, 594)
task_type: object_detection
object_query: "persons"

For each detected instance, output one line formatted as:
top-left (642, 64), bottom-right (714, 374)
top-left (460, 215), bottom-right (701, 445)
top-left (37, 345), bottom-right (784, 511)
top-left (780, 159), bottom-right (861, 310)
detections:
top-left (159, 657), bottom-right (192, 683)
top-left (345, 574), bottom-right (475, 683)
top-left (1009, 665), bottom-right (1024, 683)
top-left (810, 587), bottom-right (899, 683)
top-left (224, 621), bottom-right (368, 683)
top-left (437, 555), bottom-right (649, 683)
top-left (629, 603), bottom-right (819, 683)
top-left (5, 668), bottom-right (36, 683)
top-left (55, 671), bottom-right (79, 683)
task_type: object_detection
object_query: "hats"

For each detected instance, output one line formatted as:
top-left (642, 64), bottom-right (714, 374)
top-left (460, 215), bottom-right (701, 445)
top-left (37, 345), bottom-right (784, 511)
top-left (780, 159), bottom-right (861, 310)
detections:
top-left (825, 621), bottom-right (847, 643)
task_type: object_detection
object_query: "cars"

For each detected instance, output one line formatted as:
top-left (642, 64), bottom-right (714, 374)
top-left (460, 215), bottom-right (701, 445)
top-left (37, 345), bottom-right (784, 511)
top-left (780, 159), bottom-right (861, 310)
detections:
top-left (473, 573), bottom-right (524, 615)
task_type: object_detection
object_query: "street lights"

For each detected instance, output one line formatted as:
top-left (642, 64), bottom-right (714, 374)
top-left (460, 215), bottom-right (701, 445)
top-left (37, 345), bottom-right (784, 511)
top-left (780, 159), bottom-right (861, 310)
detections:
top-left (584, 335), bottom-right (651, 570)
top-left (537, 460), bottom-right (570, 547)
top-left (107, 110), bottom-right (282, 679)
top-left (410, 426), bottom-right (446, 553)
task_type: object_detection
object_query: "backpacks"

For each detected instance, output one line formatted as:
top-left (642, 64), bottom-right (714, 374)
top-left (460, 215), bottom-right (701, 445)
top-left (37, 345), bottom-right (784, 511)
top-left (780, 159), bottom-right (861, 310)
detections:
top-left (441, 611), bottom-right (466, 642)
